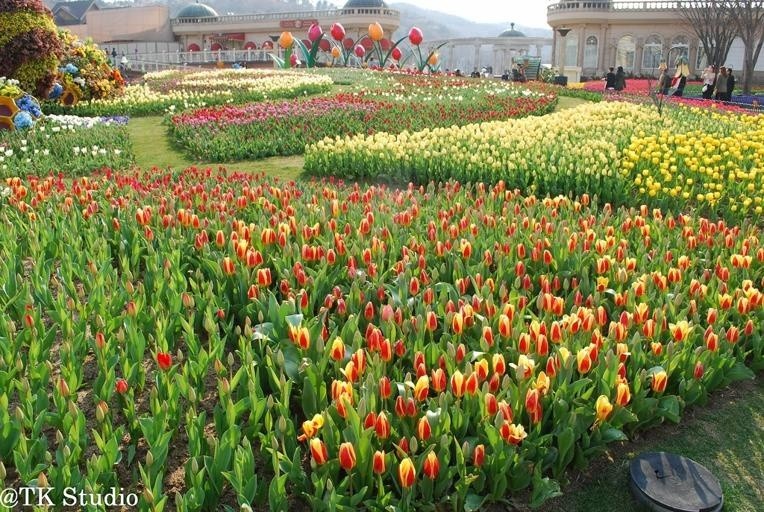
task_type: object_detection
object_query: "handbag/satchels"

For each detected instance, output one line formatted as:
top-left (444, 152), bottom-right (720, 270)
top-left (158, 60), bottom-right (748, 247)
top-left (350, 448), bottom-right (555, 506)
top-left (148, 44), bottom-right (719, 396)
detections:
top-left (701, 86), bottom-right (708, 93)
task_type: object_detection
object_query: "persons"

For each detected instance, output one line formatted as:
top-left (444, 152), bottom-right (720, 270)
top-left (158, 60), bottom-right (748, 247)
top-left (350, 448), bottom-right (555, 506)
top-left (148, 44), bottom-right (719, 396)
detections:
top-left (700, 64), bottom-right (716, 100)
top-left (614, 65), bottom-right (626, 92)
top-left (714, 66), bottom-right (729, 102)
top-left (230, 60), bottom-right (249, 70)
top-left (444, 63), bottom-right (529, 84)
top-left (110, 48), bottom-right (116, 57)
top-left (726, 68), bottom-right (735, 102)
top-left (368, 58), bottom-right (374, 68)
top-left (362, 59), bottom-right (368, 68)
top-left (599, 66), bottom-right (616, 92)
top-left (656, 65), bottom-right (672, 96)
top-left (104, 47), bottom-right (108, 54)
top-left (671, 73), bottom-right (686, 97)
